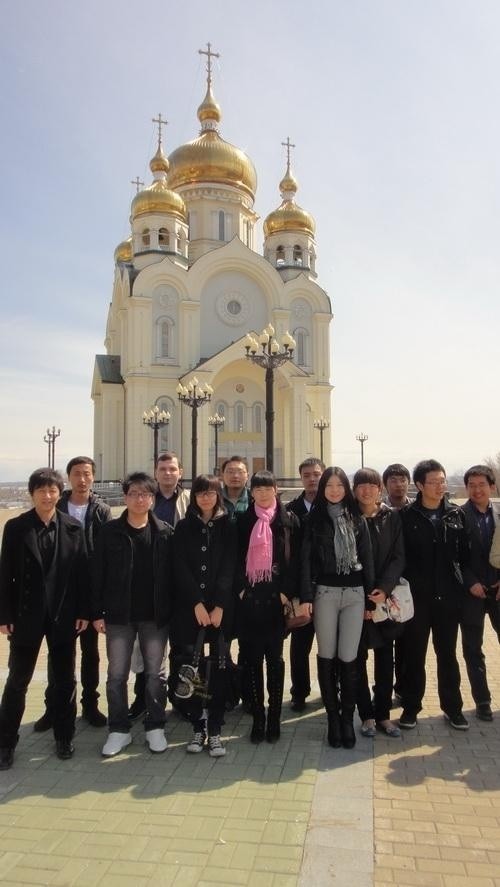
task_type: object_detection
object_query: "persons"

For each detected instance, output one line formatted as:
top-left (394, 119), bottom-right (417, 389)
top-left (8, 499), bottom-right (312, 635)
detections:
top-left (1, 455), bottom-right (500, 770)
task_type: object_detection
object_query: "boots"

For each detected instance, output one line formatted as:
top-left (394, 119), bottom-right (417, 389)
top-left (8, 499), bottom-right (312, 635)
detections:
top-left (249, 667), bottom-right (266, 746)
top-left (316, 654), bottom-right (342, 750)
top-left (265, 663), bottom-right (285, 745)
top-left (337, 657), bottom-right (360, 750)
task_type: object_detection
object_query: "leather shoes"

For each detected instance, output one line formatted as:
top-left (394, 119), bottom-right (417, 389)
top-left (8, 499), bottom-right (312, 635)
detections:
top-left (361, 709), bottom-right (417, 738)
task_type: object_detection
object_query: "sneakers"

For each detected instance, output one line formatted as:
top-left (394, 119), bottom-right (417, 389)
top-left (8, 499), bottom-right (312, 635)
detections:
top-left (476, 704), bottom-right (493, 721)
top-left (291, 699), bottom-right (306, 714)
top-left (34, 705), bottom-right (226, 760)
top-left (0, 749), bottom-right (14, 770)
top-left (444, 710), bottom-right (470, 729)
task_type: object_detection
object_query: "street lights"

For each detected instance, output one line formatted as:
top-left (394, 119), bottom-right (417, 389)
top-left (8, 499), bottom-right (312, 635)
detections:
top-left (175, 376), bottom-right (214, 488)
top-left (47, 426), bottom-right (61, 470)
top-left (209, 412), bottom-right (226, 471)
top-left (141, 405), bottom-right (172, 478)
top-left (44, 435), bottom-right (54, 467)
top-left (243, 325), bottom-right (297, 475)
top-left (312, 414), bottom-right (333, 461)
top-left (355, 432), bottom-right (369, 469)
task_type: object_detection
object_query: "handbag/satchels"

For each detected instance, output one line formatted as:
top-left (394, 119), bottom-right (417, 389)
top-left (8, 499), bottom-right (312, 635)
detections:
top-left (372, 578), bottom-right (415, 622)
top-left (168, 654), bottom-right (240, 711)
top-left (283, 594), bottom-right (313, 630)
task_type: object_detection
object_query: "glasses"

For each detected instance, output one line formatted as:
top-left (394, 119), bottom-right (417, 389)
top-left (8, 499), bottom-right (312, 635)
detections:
top-left (196, 490), bottom-right (218, 498)
top-left (127, 490), bottom-right (154, 500)
top-left (426, 479), bottom-right (448, 488)
